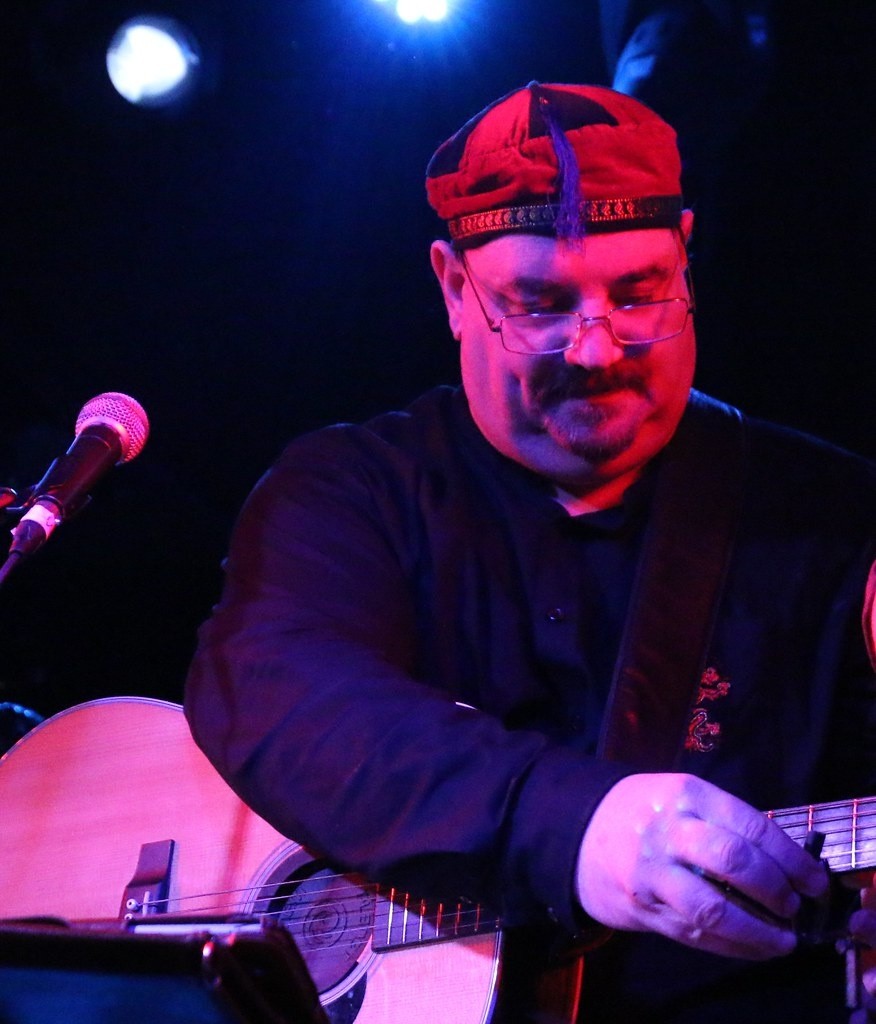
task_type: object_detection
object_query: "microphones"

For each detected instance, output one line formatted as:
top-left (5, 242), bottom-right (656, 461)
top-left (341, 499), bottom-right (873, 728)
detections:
top-left (9, 392), bottom-right (149, 556)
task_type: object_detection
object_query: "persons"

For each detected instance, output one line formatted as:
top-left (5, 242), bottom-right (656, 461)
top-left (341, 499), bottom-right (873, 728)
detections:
top-left (178, 80), bottom-right (875, 1024)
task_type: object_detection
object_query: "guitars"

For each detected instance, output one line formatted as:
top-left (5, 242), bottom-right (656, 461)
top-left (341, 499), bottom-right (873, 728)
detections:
top-left (0, 693), bottom-right (876, 1023)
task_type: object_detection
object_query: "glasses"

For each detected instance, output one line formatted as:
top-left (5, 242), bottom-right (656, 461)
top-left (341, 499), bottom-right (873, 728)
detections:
top-left (459, 226), bottom-right (694, 354)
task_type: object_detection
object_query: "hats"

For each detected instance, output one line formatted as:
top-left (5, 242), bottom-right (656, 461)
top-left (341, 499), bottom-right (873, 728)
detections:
top-left (424, 80), bottom-right (683, 249)
